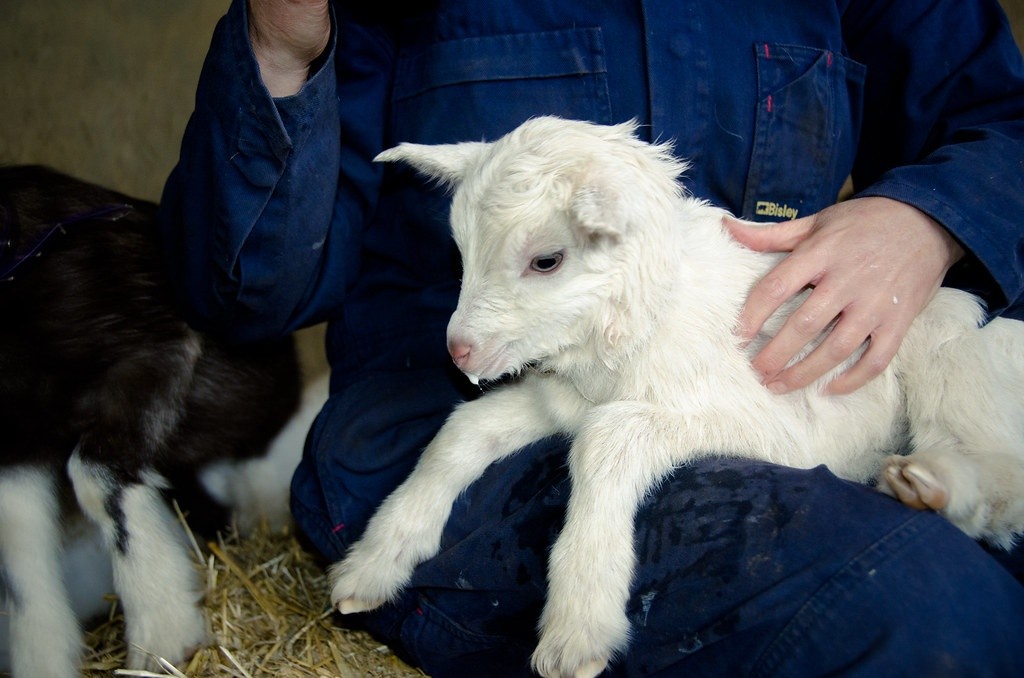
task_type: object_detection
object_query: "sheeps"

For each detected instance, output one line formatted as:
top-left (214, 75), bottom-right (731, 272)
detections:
top-left (0, 162), bottom-right (331, 677)
top-left (324, 115), bottom-right (1023, 677)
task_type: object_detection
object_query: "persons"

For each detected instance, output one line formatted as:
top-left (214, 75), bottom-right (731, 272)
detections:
top-left (158, 0), bottom-right (1024, 678)
